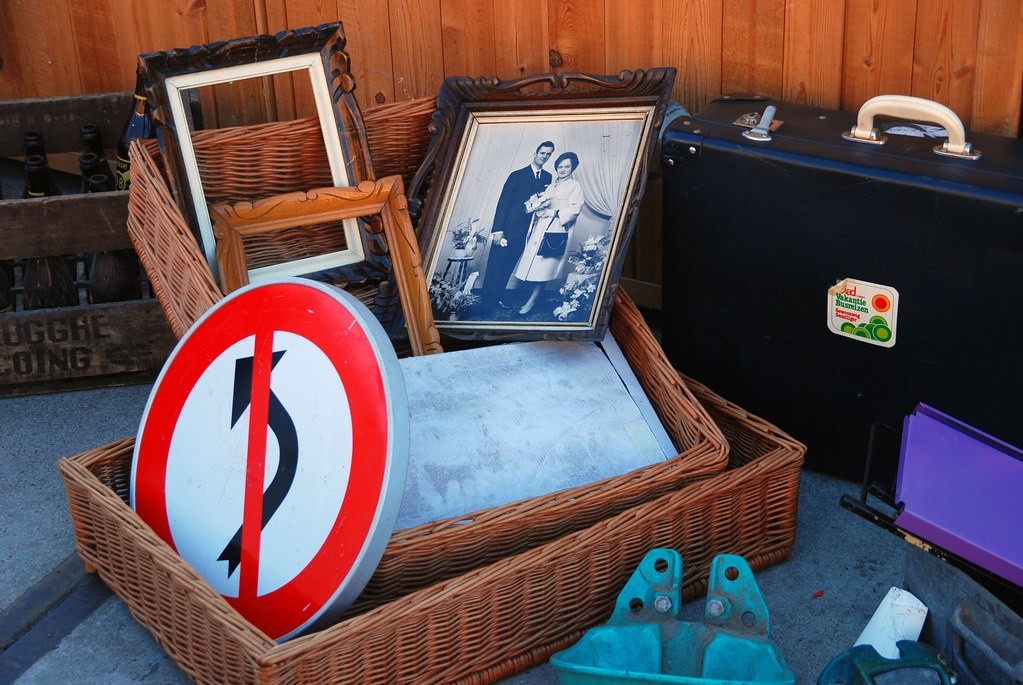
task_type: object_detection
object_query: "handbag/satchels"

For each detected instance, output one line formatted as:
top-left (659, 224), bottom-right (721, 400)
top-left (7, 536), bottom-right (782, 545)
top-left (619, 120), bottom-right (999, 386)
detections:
top-left (535, 211), bottom-right (569, 258)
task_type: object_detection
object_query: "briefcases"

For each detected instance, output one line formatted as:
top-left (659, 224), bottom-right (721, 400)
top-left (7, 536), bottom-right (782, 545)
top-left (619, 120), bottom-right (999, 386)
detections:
top-left (660, 91), bottom-right (1023, 486)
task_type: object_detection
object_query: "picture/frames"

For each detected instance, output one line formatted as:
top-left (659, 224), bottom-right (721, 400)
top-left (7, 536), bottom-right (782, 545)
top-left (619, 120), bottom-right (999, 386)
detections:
top-left (374, 67), bottom-right (680, 345)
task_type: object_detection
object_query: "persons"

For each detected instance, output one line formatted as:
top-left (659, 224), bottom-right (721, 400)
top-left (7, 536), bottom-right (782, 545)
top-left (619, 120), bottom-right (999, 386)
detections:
top-left (514, 152), bottom-right (584, 315)
top-left (479, 141), bottom-right (555, 318)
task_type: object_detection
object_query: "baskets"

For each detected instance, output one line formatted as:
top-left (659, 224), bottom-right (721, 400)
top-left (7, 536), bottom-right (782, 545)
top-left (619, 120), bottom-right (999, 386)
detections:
top-left (125, 97), bottom-right (449, 343)
top-left (334, 282), bottom-right (728, 619)
top-left (58, 367), bottom-right (804, 685)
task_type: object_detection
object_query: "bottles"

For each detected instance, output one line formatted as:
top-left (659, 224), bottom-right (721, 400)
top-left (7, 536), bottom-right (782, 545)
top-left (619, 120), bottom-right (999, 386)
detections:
top-left (0, 68), bottom-right (193, 316)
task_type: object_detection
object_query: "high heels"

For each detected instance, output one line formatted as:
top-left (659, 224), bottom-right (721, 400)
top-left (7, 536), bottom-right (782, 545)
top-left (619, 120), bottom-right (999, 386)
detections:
top-left (513, 304), bottom-right (535, 317)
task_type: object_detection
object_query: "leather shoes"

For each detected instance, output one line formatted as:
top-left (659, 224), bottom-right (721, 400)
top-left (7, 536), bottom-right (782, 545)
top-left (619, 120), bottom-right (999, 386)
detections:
top-left (497, 300), bottom-right (511, 309)
top-left (481, 307), bottom-right (492, 319)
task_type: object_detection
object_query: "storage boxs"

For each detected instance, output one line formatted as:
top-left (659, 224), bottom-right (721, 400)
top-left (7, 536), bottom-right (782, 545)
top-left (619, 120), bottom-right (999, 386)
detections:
top-left (0, 95), bottom-right (178, 397)
top-left (902, 542), bottom-right (1023, 685)
